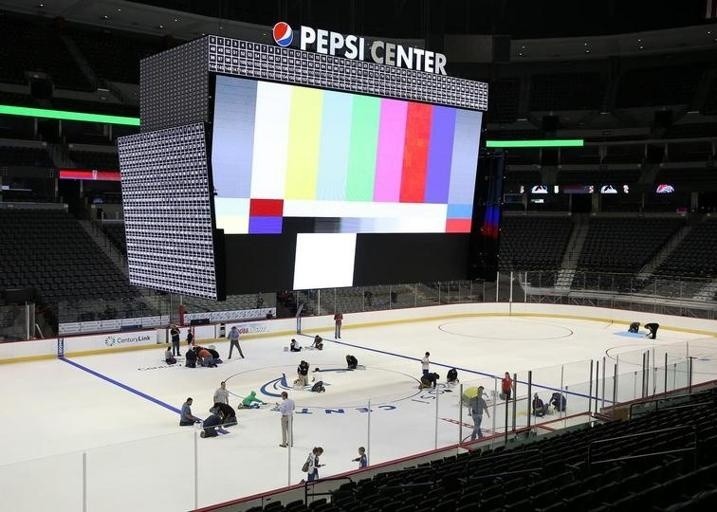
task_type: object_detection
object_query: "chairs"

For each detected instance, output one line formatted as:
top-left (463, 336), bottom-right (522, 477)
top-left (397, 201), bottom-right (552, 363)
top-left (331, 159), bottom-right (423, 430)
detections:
top-left (0, 205), bottom-right (160, 325)
top-left (161, 290), bottom-right (269, 314)
top-left (284, 281), bottom-right (482, 317)
top-left (567, 385), bottom-right (717, 511)
top-left (495, 215), bottom-right (717, 302)
top-left (237, 436), bottom-right (568, 511)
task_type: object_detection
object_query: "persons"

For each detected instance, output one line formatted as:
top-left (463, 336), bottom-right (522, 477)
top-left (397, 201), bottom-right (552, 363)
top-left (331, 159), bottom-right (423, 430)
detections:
top-left (644, 323), bottom-right (659, 339)
top-left (628, 322), bottom-right (640, 333)
top-left (256, 292), bottom-right (264, 308)
top-left (266, 311), bottom-right (274, 319)
top-left (391, 290), bottom-right (398, 303)
top-left (364, 288), bottom-right (373, 306)
top-left (418, 351), bottom-right (460, 389)
top-left (468, 372), bottom-right (566, 441)
top-left (278, 290), bottom-right (290, 307)
top-left (164, 323), bottom-right (245, 367)
top-left (276, 308), bottom-right (367, 481)
top-left (179, 381), bottom-right (268, 439)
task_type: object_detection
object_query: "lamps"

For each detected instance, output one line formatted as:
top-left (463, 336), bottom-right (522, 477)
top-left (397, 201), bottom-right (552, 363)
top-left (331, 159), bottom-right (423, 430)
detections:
top-left (651, 168), bottom-right (679, 195)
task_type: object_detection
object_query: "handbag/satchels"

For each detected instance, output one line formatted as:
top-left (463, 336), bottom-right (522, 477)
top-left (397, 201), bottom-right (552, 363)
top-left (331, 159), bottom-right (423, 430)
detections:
top-left (302, 462), bottom-right (310, 473)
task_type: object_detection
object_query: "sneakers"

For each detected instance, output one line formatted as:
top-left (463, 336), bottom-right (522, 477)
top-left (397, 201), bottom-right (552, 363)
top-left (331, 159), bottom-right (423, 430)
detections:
top-left (172, 354), bottom-right (181, 356)
top-left (335, 336), bottom-right (341, 339)
top-left (228, 356), bottom-right (244, 359)
top-left (279, 443), bottom-right (289, 448)
